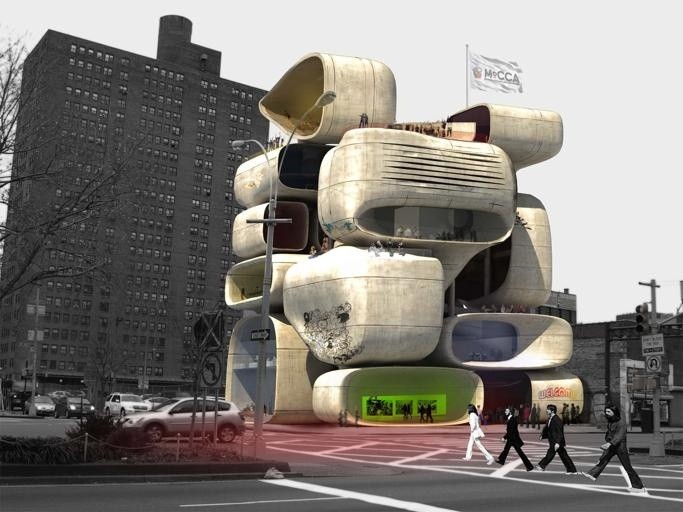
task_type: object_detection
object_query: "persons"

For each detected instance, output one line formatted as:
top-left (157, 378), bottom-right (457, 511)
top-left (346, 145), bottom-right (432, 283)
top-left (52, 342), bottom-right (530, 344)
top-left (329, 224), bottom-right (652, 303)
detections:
top-left (364, 113), bottom-right (368, 128)
top-left (536, 404), bottom-right (578, 475)
top-left (461, 403), bottom-right (494, 466)
top-left (476, 402), bottom-right (583, 433)
top-left (343, 408), bottom-right (348, 426)
top-left (404, 117), bottom-right (453, 138)
top-left (400, 402), bottom-right (434, 422)
top-left (337, 409), bottom-right (344, 427)
top-left (581, 404), bottom-right (646, 493)
top-left (358, 113), bottom-right (365, 128)
top-left (354, 409), bottom-right (361, 426)
top-left (495, 408), bottom-right (534, 472)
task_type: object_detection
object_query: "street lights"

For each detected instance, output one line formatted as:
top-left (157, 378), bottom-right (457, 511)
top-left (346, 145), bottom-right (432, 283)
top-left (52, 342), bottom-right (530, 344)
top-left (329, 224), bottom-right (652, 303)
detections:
top-left (231, 91), bottom-right (337, 460)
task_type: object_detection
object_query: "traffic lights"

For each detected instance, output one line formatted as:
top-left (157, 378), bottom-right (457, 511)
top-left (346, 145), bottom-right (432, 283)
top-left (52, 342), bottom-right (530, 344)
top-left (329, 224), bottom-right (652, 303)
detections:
top-left (635, 303), bottom-right (649, 336)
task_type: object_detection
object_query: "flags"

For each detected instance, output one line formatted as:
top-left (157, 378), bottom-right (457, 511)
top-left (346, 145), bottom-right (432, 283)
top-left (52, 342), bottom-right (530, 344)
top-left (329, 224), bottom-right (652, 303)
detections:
top-left (468, 51), bottom-right (525, 95)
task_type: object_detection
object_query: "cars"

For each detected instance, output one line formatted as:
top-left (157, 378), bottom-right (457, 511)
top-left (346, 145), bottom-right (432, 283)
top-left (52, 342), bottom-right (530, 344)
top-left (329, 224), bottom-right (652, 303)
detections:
top-left (9, 389), bottom-right (96, 419)
top-left (102, 391), bottom-right (246, 443)
